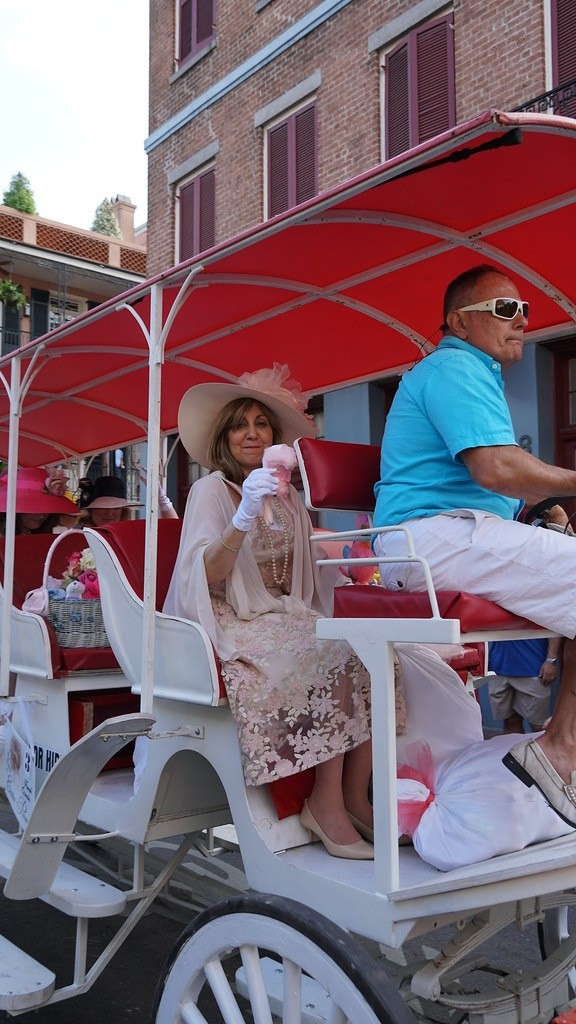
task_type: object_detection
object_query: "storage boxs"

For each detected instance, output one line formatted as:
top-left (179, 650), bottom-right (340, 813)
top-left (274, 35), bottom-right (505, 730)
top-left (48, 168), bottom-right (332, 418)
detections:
top-left (44, 528), bottom-right (108, 647)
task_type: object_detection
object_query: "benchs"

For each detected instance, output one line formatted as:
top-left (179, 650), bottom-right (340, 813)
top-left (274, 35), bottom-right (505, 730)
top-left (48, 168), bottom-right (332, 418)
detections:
top-left (0, 534), bottom-right (136, 771)
top-left (293, 438), bottom-right (564, 892)
top-left (84, 520), bottom-right (325, 850)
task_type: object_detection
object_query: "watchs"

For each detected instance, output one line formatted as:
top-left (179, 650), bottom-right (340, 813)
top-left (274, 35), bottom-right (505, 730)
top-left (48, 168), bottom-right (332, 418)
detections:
top-left (545, 657), bottom-right (558, 665)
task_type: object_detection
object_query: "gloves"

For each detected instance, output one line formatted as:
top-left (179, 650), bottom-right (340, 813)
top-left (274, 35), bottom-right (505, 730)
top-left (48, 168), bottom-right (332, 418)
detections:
top-left (140, 467), bottom-right (173, 512)
top-left (232, 469), bottom-right (279, 533)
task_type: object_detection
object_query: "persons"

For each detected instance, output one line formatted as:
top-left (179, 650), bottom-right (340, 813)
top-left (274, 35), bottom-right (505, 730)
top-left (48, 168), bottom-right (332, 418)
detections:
top-left (483, 631), bottom-right (562, 734)
top-left (159, 397), bottom-right (420, 859)
top-left (3, 470), bottom-right (67, 532)
top-left (369, 258), bottom-right (576, 831)
top-left (87, 477), bottom-right (131, 526)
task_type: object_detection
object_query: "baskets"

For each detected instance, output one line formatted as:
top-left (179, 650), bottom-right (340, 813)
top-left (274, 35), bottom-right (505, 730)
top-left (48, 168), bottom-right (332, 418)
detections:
top-left (41, 529), bottom-right (112, 648)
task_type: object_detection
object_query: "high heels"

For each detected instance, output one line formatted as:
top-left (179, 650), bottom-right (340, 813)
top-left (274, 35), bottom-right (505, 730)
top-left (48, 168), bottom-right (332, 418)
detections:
top-left (300, 799), bottom-right (414, 860)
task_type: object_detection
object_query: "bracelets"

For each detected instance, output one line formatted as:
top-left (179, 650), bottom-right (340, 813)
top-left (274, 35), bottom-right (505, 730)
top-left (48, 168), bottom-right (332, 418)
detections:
top-left (220, 532), bottom-right (240, 553)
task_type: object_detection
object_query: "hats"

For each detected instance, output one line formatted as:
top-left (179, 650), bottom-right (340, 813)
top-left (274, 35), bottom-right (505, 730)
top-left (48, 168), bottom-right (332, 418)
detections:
top-left (0, 468), bottom-right (81, 514)
top-left (177, 382), bottom-right (314, 471)
top-left (79, 476), bottom-right (145, 509)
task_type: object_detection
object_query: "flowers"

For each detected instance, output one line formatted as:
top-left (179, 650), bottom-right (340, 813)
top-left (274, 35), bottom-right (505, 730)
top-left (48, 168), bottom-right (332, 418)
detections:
top-left (51, 549), bottom-right (101, 600)
top-left (42, 470), bottom-right (69, 495)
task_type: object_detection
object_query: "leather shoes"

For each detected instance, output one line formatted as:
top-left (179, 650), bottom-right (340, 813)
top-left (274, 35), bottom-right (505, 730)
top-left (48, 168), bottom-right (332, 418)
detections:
top-left (499, 737), bottom-right (576, 828)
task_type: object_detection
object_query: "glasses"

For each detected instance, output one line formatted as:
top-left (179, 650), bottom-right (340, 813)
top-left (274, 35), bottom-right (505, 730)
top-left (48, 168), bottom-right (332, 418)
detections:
top-left (458, 298), bottom-right (528, 322)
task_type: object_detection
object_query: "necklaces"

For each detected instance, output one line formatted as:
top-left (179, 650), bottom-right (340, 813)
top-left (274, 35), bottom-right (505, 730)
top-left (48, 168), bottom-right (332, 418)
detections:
top-left (253, 493), bottom-right (291, 585)
